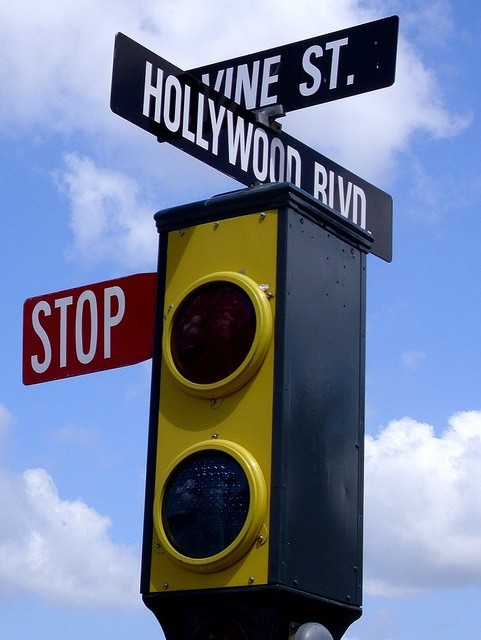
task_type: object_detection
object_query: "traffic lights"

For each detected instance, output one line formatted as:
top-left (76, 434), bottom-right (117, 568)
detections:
top-left (163, 272), bottom-right (273, 398)
top-left (153, 439), bottom-right (266, 574)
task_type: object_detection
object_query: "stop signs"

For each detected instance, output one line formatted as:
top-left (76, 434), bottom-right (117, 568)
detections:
top-left (22, 271), bottom-right (155, 388)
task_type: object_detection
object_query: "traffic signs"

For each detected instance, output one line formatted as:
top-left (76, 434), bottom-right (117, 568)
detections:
top-left (110, 31), bottom-right (393, 265)
top-left (156, 14), bottom-right (401, 143)
